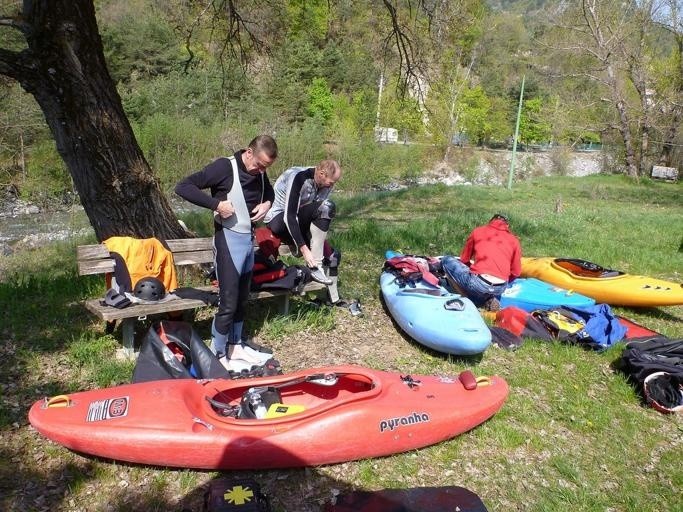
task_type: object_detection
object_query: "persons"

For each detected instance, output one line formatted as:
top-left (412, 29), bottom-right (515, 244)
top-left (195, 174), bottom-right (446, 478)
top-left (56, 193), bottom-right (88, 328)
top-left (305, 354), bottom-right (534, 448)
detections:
top-left (441, 214), bottom-right (521, 306)
top-left (172, 134), bottom-right (272, 371)
top-left (260, 160), bottom-right (348, 308)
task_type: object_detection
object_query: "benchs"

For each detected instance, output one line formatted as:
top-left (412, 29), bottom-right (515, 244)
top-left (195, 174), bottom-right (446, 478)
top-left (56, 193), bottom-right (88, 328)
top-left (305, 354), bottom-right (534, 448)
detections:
top-left (650, 165), bottom-right (679, 183)
top-left (77, 234), bottom-right (340, 360)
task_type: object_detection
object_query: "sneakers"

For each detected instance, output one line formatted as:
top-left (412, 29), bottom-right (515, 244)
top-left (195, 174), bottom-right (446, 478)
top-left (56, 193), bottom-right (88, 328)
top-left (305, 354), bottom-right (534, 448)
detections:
top-left (348, 300), bottom-right (365, 318)
top-left (307, 265), bottom-right (333, 287)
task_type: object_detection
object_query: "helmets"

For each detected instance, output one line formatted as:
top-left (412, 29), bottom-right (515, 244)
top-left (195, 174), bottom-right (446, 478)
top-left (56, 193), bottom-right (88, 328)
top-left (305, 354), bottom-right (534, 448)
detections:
top-left (134, 275), bottom-right (167, 301)
top-left (642, 370), bottom-right (683, 415)
top-left (255, 226), bottom-right (282, 257)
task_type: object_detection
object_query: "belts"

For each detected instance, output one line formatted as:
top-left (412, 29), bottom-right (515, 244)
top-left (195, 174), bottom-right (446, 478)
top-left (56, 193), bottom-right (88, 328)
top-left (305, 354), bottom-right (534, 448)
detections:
top-left (478, 272), bottom-right (506, 289)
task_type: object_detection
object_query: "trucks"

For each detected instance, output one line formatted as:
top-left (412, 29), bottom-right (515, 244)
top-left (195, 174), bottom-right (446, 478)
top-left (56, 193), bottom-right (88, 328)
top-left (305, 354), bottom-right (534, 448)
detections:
top-left (378, 127), bottom-right (398, 144)
